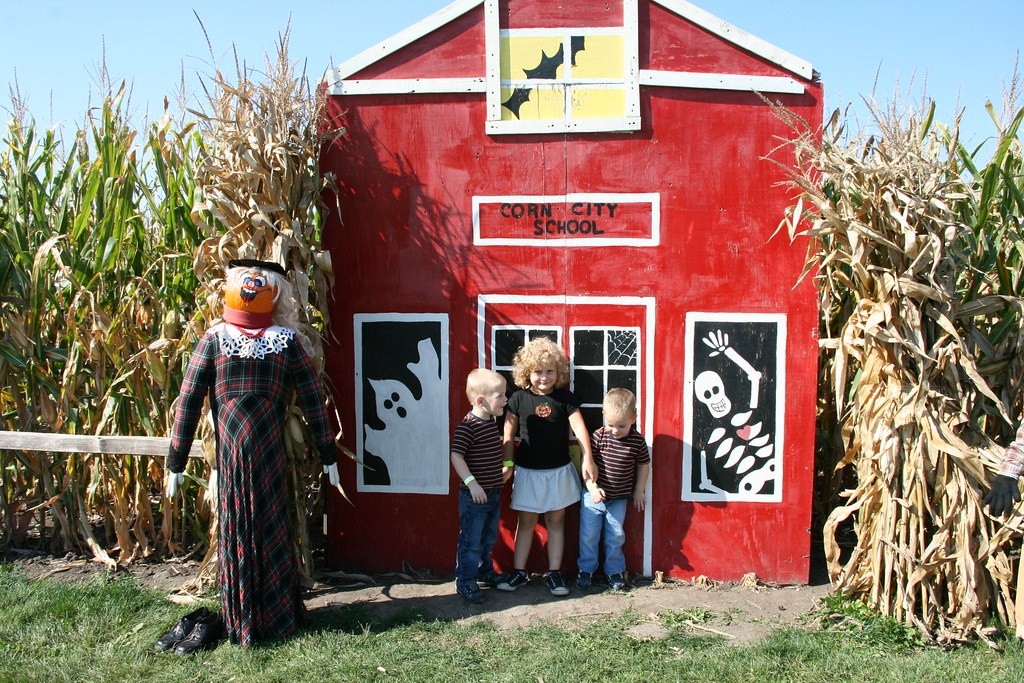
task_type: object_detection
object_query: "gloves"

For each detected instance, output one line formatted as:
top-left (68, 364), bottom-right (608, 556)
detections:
top-left (324, 461), bottom-right (340, 485)
top-left (165, 471), bottom-right (185, 498)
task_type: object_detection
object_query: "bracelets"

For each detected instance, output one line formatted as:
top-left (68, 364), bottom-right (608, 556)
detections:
top-left (501, 460), bottom-right (515, 467)
top-left (463, 475), bottom-right (476, 486)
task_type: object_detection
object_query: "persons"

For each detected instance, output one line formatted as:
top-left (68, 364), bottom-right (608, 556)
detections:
top-left (451, 367), bottom-right (509, 606)
top-left (159, 256), bottom-right (341, 650)
top-left (573, 386), bottom-right (651, 594)
top-left (495, 335), bottom-right (599, 595)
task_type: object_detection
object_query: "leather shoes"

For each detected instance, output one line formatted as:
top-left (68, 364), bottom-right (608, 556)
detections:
top-left (154, 607), bottom-right (226, 656)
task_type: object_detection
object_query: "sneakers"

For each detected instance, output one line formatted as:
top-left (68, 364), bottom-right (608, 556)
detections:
top-left (576, 569), bottom-right (594, 590)
top-left (606, 573), bottom-right (625, 591)
top-left (496, 570), bottom-right (527, 591)
top-left (478, 569), bottom-right (511, 586)
top-left (454, 581), bottom-right (485, 603)
top-left (546, 572), bottom-right (569, 595)
